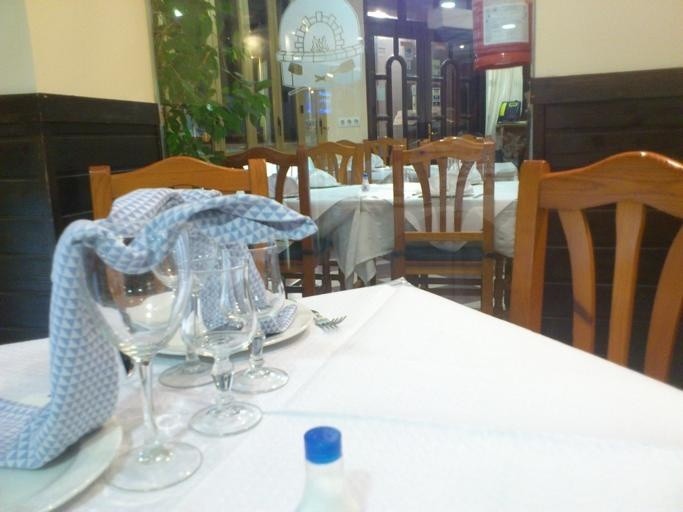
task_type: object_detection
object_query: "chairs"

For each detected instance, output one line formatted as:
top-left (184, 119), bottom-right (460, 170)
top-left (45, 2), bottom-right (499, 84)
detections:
top-left (89, 156), bottom-right (271, 306)
top-left (508, 150), bottom-right (683, 384)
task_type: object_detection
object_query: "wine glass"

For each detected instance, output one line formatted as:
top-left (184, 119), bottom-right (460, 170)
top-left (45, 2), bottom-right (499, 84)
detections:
top-left (185, 260), bottom-right (256, 437)
top-left (157, 210), bottom-right (218, 388)
top-left (219, 243), bottom-right (291, 392)
top-left (84, 231), bottom-right (203, 491)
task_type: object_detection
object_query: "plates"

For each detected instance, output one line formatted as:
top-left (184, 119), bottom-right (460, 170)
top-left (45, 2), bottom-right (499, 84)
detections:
top-left (0, 394), bottom-right (122, 512)
top-left (260, 299), bottom-right (312, 353)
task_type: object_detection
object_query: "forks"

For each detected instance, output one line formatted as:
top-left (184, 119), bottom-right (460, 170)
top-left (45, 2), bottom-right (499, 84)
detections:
top-left (310, 300), bottom-right (345, 328)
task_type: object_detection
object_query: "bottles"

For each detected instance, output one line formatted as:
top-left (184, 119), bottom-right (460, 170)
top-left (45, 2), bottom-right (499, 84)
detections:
top-left (361, 173), bottom-right (370, 199)
top-left (297, 425), bottom-right (365, 512)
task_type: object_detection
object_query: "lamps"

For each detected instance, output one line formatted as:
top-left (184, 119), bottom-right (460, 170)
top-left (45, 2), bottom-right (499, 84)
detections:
top-left (472, 0), bottom-right (532, 70)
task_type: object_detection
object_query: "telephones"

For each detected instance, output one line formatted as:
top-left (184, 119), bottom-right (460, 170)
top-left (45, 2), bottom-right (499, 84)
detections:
top-left (497, 101), bottom-right (521, 123)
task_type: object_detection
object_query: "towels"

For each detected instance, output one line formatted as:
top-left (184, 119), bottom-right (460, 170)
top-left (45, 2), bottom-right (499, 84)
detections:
top-left (1, 187), bottom-right (320, 472)
